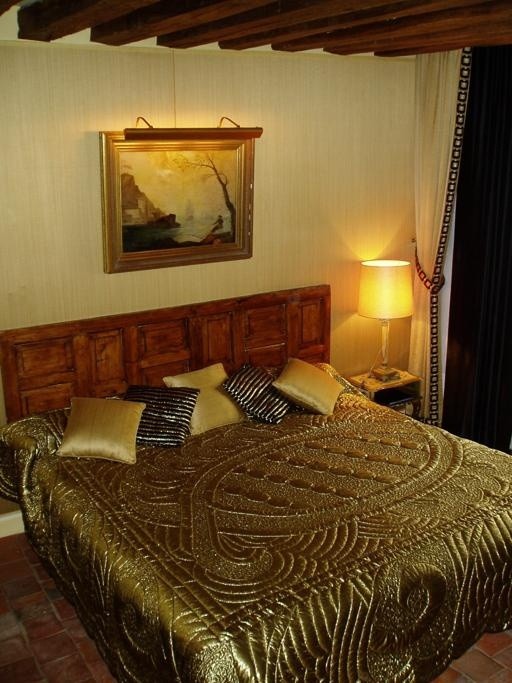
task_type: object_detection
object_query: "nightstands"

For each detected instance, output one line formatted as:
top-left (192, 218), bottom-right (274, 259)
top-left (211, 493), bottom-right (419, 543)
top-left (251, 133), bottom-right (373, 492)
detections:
top-left (347, 368), bottom-right (423, 423)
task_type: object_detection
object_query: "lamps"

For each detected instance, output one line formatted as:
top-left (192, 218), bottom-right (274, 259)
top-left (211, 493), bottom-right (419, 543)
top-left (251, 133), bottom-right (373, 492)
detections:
top-left (356, 259), bottom-right (413, 380)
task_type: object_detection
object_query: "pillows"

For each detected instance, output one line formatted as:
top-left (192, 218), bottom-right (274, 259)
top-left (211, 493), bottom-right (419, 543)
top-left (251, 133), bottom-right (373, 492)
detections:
top-left (162, 362), bottom-right (246, 436)
top-left (271, 357), bottom-right (345, 415)
top-left (124, 384), bottom-right (200, 448)
top-left (223, 361), bottom-right (291, 424)
top-left (57, 396), bottom-right (145, 464)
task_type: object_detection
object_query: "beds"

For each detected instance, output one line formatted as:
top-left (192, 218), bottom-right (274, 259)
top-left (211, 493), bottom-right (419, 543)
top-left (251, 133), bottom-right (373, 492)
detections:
top-left (0, 286), bottom-right (512, 683)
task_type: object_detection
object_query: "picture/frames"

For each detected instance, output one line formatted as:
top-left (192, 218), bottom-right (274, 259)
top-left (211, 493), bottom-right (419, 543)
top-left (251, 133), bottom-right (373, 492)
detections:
top-left (99, 130), bottom-right (256, 275)
